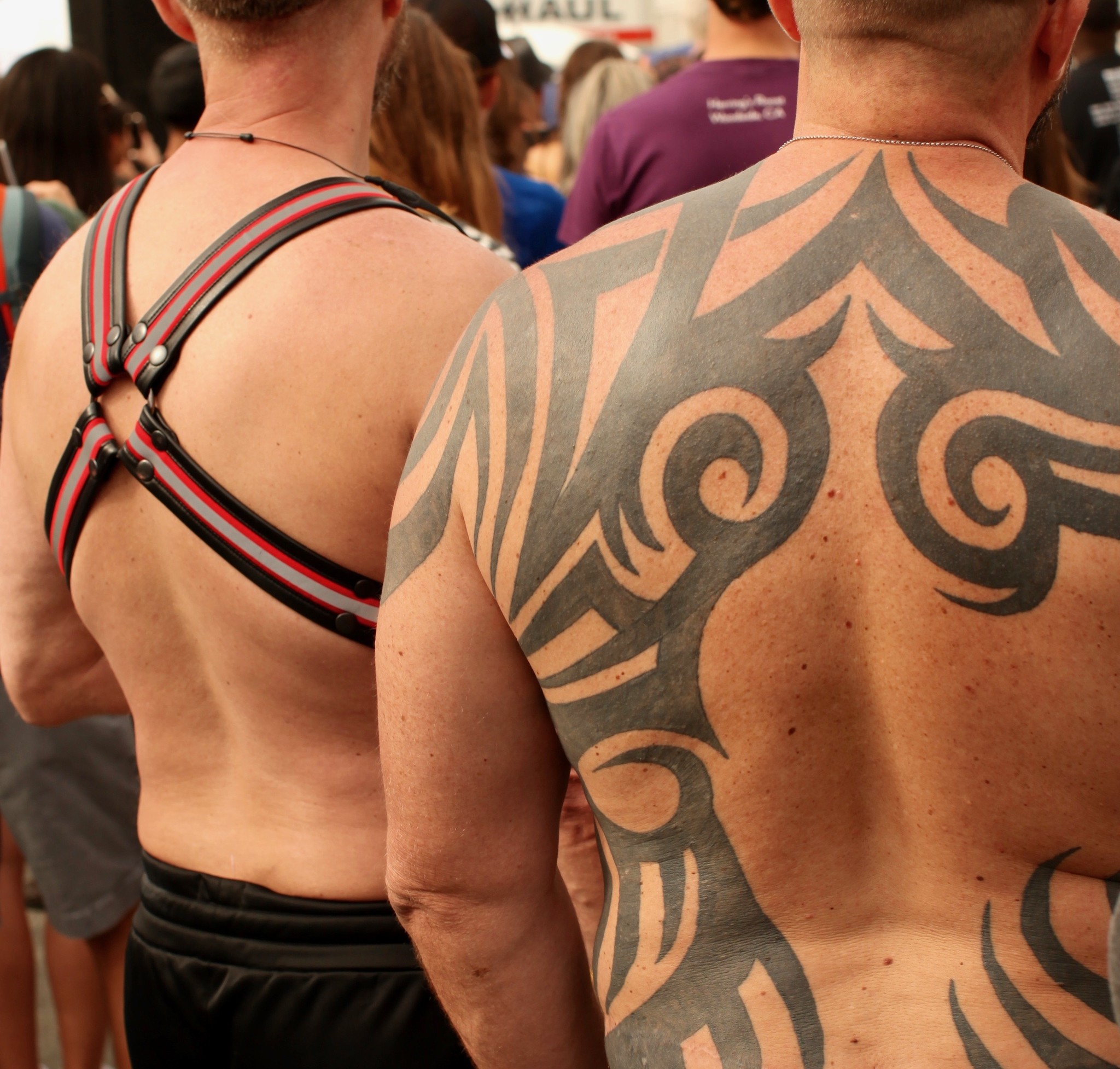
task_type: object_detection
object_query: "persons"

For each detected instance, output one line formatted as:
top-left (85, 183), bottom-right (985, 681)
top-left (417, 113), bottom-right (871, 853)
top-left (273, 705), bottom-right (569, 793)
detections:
top-left (0, 94), bottom-right (144, 1068)
top-left (371, 1), bottom-right (1120, 1069)
top-left (0, 0), bottom-right (608, 1069)
top-left (0, 2), bottom-right (1120, 268)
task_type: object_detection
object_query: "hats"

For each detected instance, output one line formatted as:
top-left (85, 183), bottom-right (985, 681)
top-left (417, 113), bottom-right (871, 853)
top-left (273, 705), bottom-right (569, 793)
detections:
top-left (507, 37), bottom-right (553, 88)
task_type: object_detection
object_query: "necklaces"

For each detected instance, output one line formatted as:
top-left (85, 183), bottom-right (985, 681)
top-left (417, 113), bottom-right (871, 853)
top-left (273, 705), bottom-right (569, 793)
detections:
top-left (182, 129), bottom-right (465, 232)
